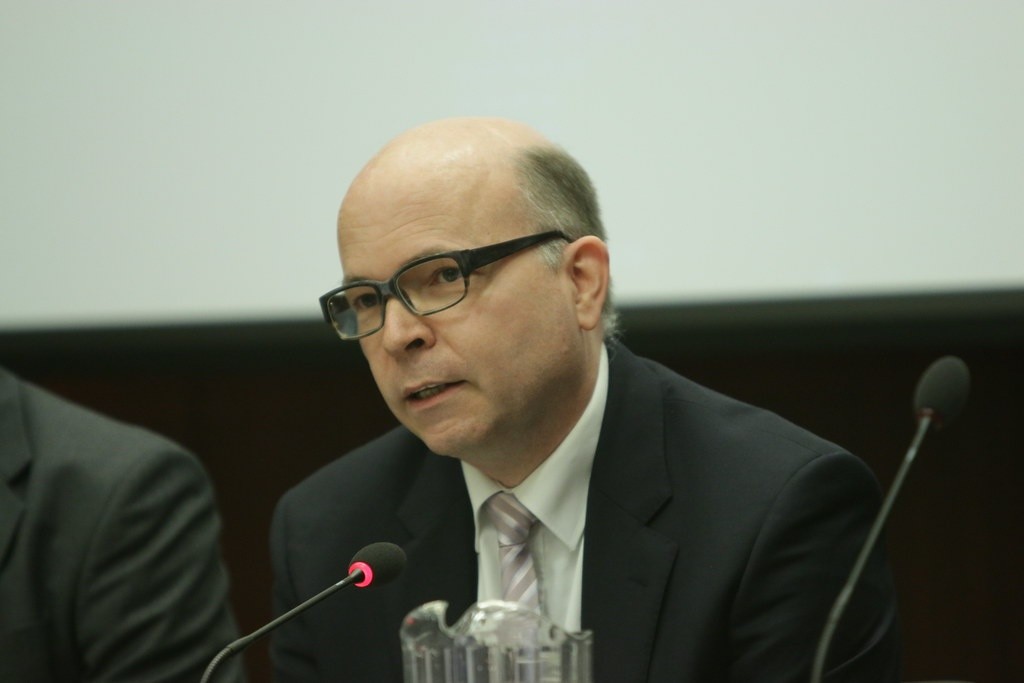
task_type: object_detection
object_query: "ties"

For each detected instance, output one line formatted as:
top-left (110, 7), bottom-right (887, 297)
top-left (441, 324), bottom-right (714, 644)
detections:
top-left (484, 492), bottom-right (542, 683)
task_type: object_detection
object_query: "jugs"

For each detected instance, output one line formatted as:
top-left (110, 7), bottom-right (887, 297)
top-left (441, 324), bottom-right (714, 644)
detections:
top-left (400, 600), bottom-right (593, 683)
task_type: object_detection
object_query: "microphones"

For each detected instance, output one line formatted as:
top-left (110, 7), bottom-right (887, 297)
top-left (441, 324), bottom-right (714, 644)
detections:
top-left (200, 542), bottom-right (406, 683)
top-left (808, 356), bottom-right (970, 683)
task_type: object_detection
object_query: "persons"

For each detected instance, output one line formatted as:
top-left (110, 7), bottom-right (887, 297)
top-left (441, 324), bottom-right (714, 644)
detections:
top-left (265, 119), bottom-right (884, 683)
top-left (0, 371), bottom-right (244, 683)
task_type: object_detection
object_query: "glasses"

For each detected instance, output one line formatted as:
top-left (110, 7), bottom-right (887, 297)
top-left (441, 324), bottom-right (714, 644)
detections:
top-left (319, 231), bottom-right (572, 342)
